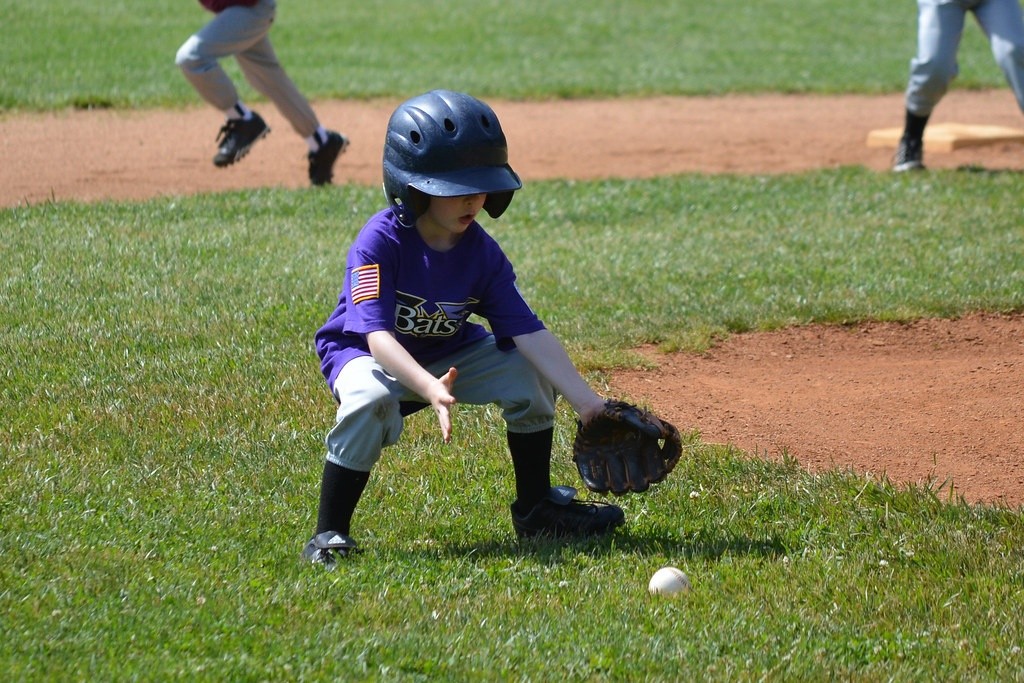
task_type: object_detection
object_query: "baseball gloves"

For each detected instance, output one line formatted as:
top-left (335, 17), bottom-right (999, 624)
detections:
top-left (572, 401), bottom-right (684, 496)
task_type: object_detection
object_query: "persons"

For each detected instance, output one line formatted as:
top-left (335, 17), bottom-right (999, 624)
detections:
top-left (890, 0), bottom-right (1024, 172)
top-left (174, 0), bottom-right (351, 185)
top-left (301, 89), bottom-right (683, 570)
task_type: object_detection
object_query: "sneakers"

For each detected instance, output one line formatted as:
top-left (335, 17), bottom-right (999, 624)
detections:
top-left (510, 484), bottom-right (623, 541)
top-left (211, 111), bottom-right (271, 167)
top-left (309, 130), bottom-right (352, 187)
top-left (894, 135), bottom-right (922, 171)
top-left (302, 529), bottom-right (356, 567)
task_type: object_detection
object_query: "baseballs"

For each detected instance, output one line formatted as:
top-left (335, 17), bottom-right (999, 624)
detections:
top-left (648, 566), bottom-right (690, 598)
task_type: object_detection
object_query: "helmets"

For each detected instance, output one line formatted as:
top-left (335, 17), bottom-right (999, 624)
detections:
top-left (381, 89), bottom-right (522, 227)
